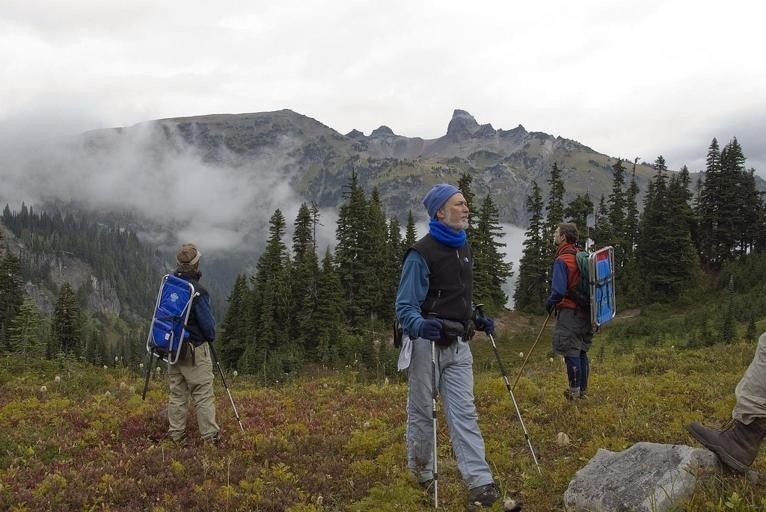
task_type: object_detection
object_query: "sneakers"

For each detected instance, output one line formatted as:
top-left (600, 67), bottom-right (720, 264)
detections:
top-left (564, 389), bottom-right (587, 400)
top-left (420, 479), bottom-right (435, 493)
top-left (468, 485), bottom-right (522, 512)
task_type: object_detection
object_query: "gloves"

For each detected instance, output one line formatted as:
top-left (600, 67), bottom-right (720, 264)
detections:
top-left (420, 318), bottom-right (443, 341)
top-left (475, 312), bottom-right (495, 335)
top-left (546, 299), bottom-right (554, 313)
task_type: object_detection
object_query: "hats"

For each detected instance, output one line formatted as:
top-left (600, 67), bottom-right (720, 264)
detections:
top-left (423, 183), bottom-right (463, 220)
top-left (176, 244), bottom-right (199, 266)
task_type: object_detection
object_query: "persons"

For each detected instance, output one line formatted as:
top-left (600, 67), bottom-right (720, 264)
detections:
top-left (543, 223), bottom-right (594, 402)
top-left (395, 183), bottom-right (525, 512)
top-left (161, 243), bottom-right (227, 451)
top-left (686, 330), bottom-right (764, 477)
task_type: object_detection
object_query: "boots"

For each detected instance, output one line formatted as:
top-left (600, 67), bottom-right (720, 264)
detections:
top-left (686, 416), bottom-right (765, 475)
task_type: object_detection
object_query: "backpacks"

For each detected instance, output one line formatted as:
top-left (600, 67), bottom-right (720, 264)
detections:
top-left (555, 250), bottom-right (589, 311)
